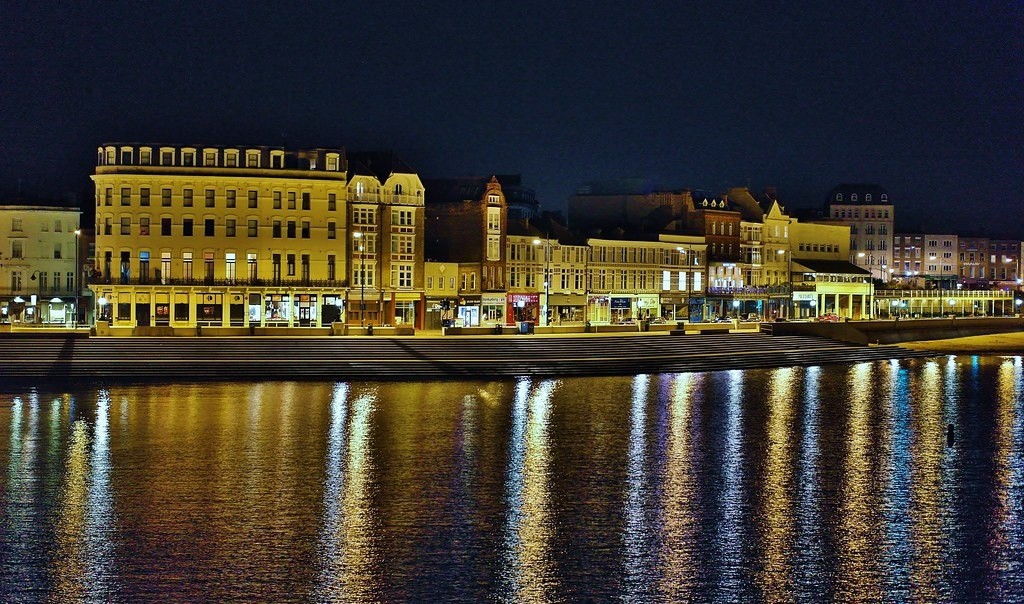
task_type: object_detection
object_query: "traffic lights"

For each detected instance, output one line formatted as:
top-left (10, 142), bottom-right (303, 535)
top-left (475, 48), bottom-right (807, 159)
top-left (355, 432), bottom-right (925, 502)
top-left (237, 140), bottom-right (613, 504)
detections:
top-left (439, 296), bottom-right (450, 312)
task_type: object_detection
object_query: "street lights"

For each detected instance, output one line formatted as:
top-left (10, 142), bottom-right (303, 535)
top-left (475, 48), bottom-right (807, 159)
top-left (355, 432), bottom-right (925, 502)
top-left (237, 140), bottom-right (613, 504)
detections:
top-left (858, 253), bottom-right (872, 321)
top-left (881, 264), bottom-right (888, 280)
top-left (99, 295), bottom-right (105, 316)
top-left (677, 246), bottom-right (692, 323)
top-left (353, 232), bottom-right (365, 327)
top-left (778, 249), bottom-right (792, 320)
top-left (929, 256), bottom-right (944, 318)
top-left (30, 268), bottom-right (42, 318)
top-left (1007, 254), bottom-right (1019, 281)
top-left (74, 226), bottom-right (81, 321)
top-left (533, 239), bottom-right (550, 326)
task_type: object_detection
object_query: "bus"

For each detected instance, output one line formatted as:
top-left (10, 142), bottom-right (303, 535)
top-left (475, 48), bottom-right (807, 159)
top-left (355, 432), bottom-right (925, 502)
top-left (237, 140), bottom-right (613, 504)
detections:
top-left (571, 310), bottom-right (584, 321)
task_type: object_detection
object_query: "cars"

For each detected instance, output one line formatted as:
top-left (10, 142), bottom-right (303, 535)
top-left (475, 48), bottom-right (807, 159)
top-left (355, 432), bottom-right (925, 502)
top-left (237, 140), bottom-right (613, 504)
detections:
top-left (714, 315), bottom-right (732, 323)
top-left (820, 312), bottom-right (839, 319)
top-left (773, 317), bottom-right (791, 323)
top-left (619, 318), bottom-right (644, 326)
top-left (650, 316), bottom-right (667, 325)
top-left (740, 318), bottom-right (761, 322)
